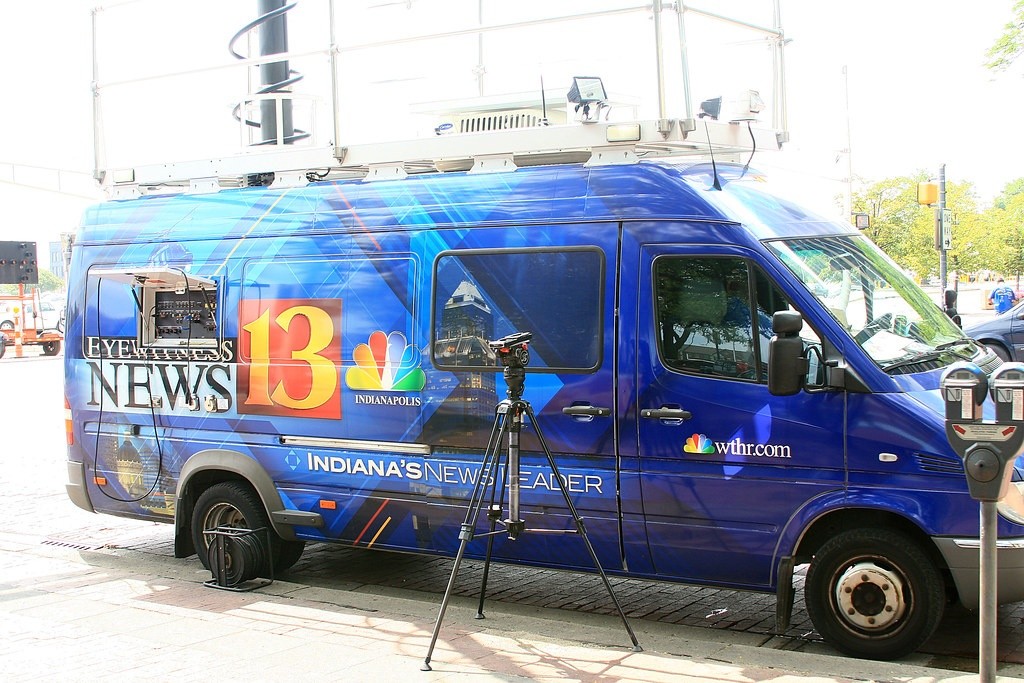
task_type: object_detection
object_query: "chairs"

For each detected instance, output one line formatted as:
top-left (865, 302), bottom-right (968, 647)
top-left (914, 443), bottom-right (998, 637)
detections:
top-left (670, 271), bottom-right (727, 360)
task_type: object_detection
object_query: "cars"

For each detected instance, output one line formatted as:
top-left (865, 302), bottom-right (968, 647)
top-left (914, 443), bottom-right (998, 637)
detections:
top-left (961, 300), bottom-right (1024, 364)
top-left (24, 292), bottom-right (64, 334)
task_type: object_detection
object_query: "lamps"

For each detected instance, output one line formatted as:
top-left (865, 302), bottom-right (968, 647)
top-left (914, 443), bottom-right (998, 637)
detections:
top-left (567, 75), bottom-right (608, 124)
top-left (698, 98), bottom-right (722, 120)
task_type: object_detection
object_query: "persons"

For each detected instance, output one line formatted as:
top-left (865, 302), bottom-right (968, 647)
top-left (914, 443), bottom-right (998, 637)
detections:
top-left (987, 275), bottom-right (1016, 317)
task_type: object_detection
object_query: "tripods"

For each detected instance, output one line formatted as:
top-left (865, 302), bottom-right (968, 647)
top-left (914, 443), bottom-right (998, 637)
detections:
top-left (419, 331), bottom-right (645, 672)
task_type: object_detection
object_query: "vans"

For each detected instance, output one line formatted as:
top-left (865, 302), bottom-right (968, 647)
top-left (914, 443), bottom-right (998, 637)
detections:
top-left (63, 75), bottom-right (1024, 659)
